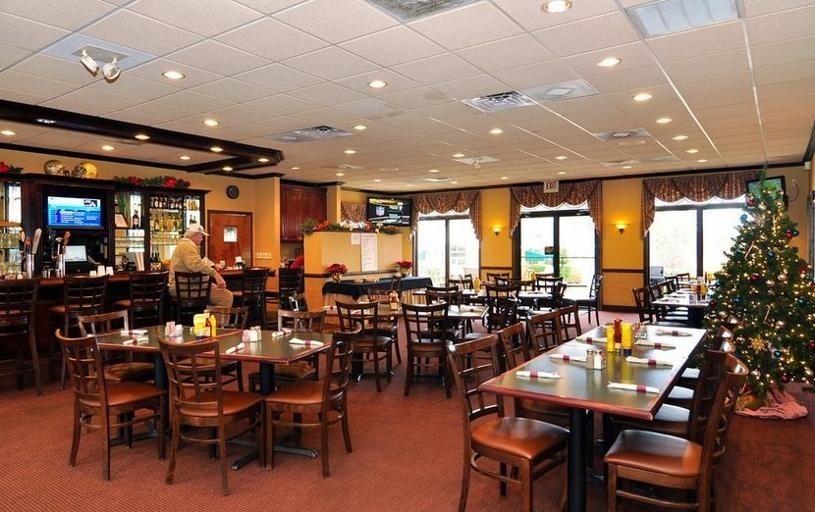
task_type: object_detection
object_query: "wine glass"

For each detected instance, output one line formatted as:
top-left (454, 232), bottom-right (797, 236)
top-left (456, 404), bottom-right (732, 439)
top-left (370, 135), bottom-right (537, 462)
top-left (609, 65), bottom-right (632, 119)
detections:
top-left (219, 260), bottom-right (226, 270)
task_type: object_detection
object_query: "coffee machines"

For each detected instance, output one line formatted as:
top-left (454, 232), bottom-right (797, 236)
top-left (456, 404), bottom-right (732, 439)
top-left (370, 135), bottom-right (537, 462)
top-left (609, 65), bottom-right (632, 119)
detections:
top-left (123, 253), bottom-right (136, 273)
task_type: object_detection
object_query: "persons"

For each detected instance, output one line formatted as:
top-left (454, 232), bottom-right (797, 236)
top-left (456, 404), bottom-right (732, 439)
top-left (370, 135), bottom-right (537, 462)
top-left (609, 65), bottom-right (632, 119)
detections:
top-left (168, 223), bottom-right (234, 327)
top-left (131, 213), bottom-right (140, 230)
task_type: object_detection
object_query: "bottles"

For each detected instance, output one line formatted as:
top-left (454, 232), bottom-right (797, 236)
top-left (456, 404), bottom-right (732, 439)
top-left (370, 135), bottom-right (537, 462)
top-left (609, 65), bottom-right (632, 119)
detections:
top-left (606, 317), bottom-right (643, 357)
top-left (166, 337), bottom-right (184, 345)
top-left (431, 299), bottom-right (444, 315)
top-left (687, 272), bottom-right (713, 304)
top-left (165, 322), bottom-right (184, 337)
top-left (606, 351), bottom-right (635, 385)
top-left (0, 231), bottom-right (22, 250)
top-left (149, 251), bottom-right (162, 272)
top-left (242, 342), bottom-right (262, 355)
top-left (41, 267), bottom-right (61, 279)
top-left (189, 308), bottom-right (217, 339)
top-left (390, 293), bottom-right (406, 311)
top-left (149, 196), bottom-right (199, 246)
top-left (472, 276), bottom-right (486, 296)
top-left (133, 210), bottom-right (140, 228)
top-left (242, 325), bottom-right (262, 341)
top-left (583, 348), bottom-right (605, 371)
top-left (530, 271), bottom-right (536, 281)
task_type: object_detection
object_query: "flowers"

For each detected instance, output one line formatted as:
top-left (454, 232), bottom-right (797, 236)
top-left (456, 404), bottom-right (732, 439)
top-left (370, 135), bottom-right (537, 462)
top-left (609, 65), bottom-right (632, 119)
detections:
top-left (395, 260), bottom-right (412, 269)
top-left (326, 264), bottom-right (348, 274)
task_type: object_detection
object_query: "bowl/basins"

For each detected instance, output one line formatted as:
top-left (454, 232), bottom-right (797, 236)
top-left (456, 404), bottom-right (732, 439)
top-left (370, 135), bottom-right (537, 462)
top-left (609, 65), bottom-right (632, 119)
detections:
top-left (351, 275), bottom-right (380, 284)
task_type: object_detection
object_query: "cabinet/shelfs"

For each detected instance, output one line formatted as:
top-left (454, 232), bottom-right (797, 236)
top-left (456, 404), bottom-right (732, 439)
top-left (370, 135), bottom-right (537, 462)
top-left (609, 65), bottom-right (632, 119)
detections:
top-left (280, 183), bottom-right (327, 243)
top-left (20, 173), bottom-right (213, 273)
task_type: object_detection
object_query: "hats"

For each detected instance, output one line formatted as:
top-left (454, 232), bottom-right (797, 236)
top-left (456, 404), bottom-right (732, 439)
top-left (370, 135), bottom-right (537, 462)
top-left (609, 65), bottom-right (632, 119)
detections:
top-left (189, 223), bottom-right (209, 237)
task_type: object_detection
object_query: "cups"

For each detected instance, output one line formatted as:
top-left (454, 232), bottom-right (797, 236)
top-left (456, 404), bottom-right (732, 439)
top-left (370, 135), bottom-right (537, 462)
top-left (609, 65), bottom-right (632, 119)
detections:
top-left (89, 265), bottom-right (113, 278)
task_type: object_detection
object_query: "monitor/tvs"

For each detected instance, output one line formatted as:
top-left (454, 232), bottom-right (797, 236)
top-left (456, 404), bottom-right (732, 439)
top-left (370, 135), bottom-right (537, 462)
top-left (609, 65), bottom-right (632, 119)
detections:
top-left (366, 196), bottom-right (412, 226)
top-left (746, 175), bottom-right (788, 211)
top-left (46, 194), bottom-right (104, 230)
